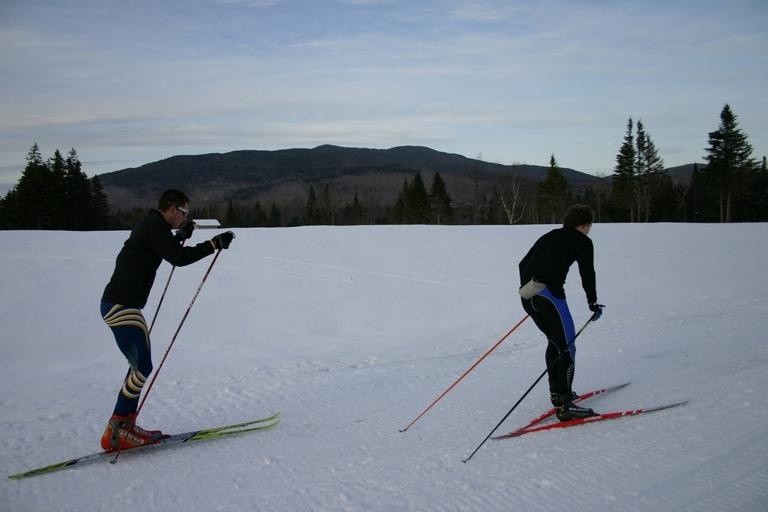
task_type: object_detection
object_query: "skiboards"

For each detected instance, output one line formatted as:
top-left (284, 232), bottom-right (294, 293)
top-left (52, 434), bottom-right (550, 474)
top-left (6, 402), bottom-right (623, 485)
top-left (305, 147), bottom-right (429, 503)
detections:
top-left (7, 411), bottom-right (280, 479)
top-left (490, 378), bottom-right (687, 440)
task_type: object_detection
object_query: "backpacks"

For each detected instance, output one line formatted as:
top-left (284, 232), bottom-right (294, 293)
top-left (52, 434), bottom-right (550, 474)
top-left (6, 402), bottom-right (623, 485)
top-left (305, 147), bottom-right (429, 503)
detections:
top-left (589, 303), bottom-right (602, 321)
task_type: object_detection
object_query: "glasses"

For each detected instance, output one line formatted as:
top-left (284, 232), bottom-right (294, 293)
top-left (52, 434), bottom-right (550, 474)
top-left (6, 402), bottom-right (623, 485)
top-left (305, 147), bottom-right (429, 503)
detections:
top-left (178, 207), bottom-right (190, 216)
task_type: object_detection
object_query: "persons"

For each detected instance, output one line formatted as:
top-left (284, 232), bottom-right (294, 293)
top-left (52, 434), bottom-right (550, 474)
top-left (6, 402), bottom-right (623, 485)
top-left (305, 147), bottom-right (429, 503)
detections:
top-left (97, 188), bottom-right (234, 449)
top-left (518, 203), bottom-right (602, 420)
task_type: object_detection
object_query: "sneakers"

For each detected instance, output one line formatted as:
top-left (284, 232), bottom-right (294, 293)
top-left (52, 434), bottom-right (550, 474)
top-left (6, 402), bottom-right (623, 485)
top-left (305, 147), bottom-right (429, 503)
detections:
top-left (101, 414), bottom-right (161, 448)
top-left (550, 390), bottom-right (593, 421)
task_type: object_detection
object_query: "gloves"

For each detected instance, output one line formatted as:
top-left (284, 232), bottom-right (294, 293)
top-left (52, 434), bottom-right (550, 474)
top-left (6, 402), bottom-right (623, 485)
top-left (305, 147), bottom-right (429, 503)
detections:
top-left (179, 221), bottom-right (196, 238)
top-left (212, 233), bottom-right (233, 249)
